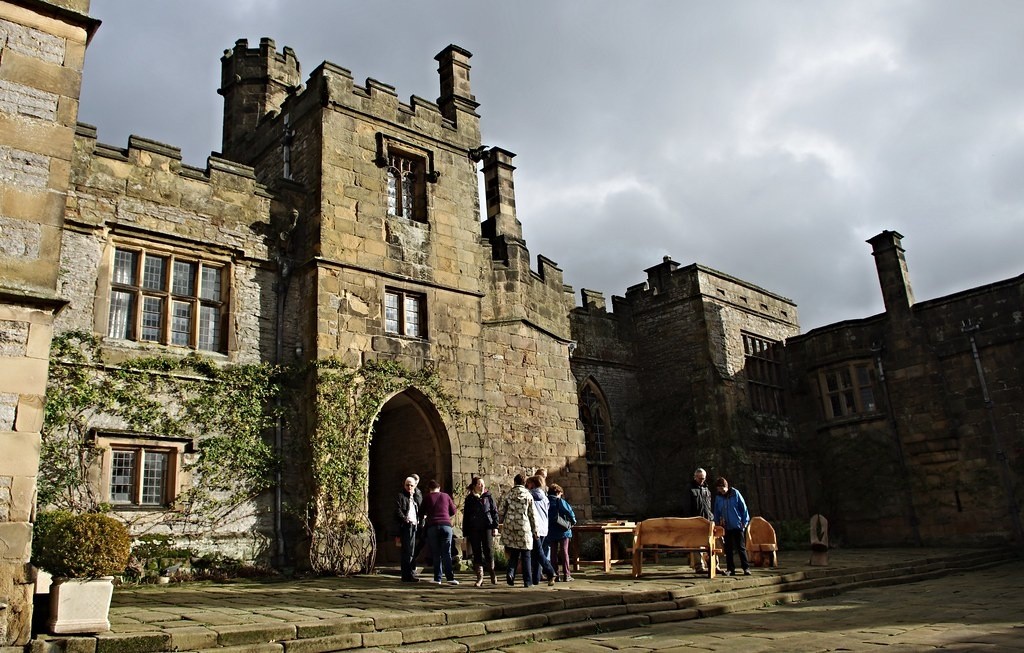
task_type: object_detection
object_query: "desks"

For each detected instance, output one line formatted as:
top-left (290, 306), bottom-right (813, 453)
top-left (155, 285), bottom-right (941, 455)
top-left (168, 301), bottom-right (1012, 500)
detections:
top-left (572, 525), bottom-right (641, 574)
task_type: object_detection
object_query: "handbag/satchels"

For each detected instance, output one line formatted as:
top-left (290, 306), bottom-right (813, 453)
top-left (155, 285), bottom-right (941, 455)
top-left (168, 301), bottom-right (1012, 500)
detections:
top-left (555, 513), bottom-right (570, 532)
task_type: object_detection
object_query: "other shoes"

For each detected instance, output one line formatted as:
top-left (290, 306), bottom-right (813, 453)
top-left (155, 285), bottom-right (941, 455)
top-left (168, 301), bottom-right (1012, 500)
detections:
top-left (401, 577), bottom-right (419, 582)
top-left (524, 582), bottom-right (529, 587)
top-left (533, 582), bottom-right (539, 585)
top-left (548, 577), bottom-right (556, 585)
top-left (726, 570), bottom-right (735, 575)
top-left (506, 574), bottom-right (514, 586)
top-left (696, 568), bottom-right (708, 574)
top-left (410, 561), bottom-right (416, 570)
top-left (540, 578), bottom-right (547, 581)
top-left (430, 580), bottom-right (441, 584)
top-left (744, 570), bottom-right (751, 575)
top-left (447, 579), bottom-right (459, 585)
top-left (716, 567), bottom-right (724, 573)
top-left (564, 576), bottom-right (574, 582)
top-left (556, 577), bottom-right (562, 581)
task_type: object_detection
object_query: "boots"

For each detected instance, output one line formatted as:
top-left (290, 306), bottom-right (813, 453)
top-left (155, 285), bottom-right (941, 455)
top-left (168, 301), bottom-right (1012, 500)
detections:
top-left (475, 566), bottom-right (483, 587)
top-left (488, 561), bottom-right (498, 584)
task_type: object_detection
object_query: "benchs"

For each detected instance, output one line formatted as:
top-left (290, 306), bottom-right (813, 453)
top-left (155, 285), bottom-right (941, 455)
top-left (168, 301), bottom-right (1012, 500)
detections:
top-left (631, 516), bottom-right (725, 578)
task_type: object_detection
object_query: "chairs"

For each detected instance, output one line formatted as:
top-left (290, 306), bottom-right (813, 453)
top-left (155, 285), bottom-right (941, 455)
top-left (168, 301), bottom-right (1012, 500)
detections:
top-left (745, 516), bottom-right (778, 568)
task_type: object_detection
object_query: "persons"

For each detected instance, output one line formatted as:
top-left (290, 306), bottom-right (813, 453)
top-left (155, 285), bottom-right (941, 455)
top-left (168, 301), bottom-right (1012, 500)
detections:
top-left (462, 477), bottom-right (500, 587)
top-left (683, 468), bottom-right (726, 574)
top-left (499, 470), bottom-right (577, 587)
top-left (713, 477), bottom-right (752, 575)
top-left (393, 474), bottom-right (459, 585)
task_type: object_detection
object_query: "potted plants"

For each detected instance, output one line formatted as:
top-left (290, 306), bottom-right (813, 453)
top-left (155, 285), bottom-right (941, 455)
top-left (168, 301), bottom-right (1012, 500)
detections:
top-left (31, 509), bottom-right (132, 633)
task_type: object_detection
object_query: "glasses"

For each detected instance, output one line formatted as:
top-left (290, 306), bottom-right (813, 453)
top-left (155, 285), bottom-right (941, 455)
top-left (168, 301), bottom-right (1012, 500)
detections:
top-left (407, 485), bottom-right (413, 487)
top-left (700, 478), bottom-right (704, 480)
